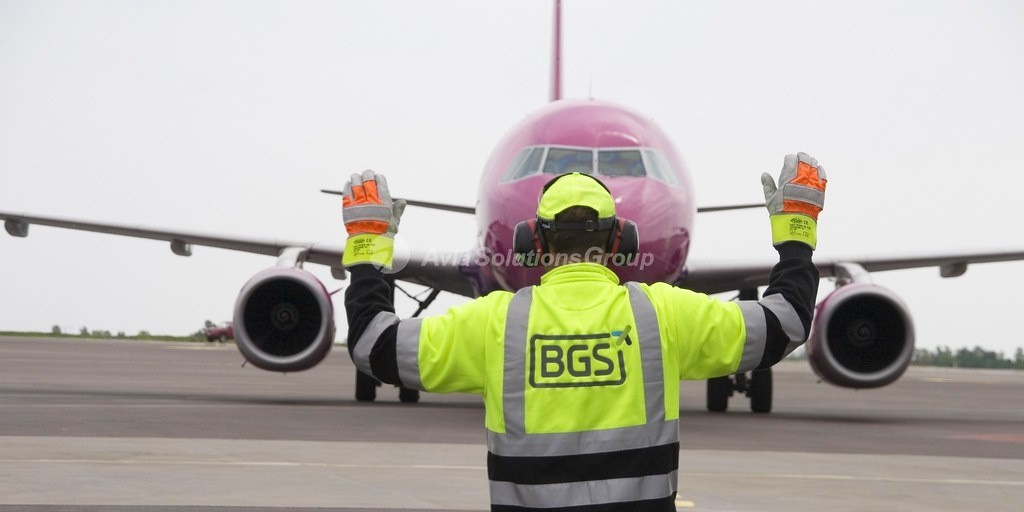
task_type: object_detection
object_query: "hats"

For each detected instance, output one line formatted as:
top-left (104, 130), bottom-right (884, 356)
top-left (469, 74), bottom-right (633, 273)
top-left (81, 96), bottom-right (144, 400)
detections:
top-left (536, 170), bottom-right (619, 232)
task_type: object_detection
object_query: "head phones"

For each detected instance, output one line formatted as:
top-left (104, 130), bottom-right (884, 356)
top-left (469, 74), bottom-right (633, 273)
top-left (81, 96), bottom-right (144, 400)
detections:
top-left (513, 172), bottom-right (640, 267)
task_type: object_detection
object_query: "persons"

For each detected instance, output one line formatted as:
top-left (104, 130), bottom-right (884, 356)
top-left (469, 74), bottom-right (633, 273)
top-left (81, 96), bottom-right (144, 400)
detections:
top-left (339, 152), bottom-right (830, 512)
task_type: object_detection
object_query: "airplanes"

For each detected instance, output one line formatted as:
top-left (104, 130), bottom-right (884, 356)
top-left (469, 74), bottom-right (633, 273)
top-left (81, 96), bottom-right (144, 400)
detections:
top-left (0, 0), bottom-right (1024, 416)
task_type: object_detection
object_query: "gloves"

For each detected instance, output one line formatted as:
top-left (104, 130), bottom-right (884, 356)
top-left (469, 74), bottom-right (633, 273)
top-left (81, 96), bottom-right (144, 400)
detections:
top-left (340, 168), bottom-right (407, 271)
top-left (760, 152), bottom-right (827, 251)
